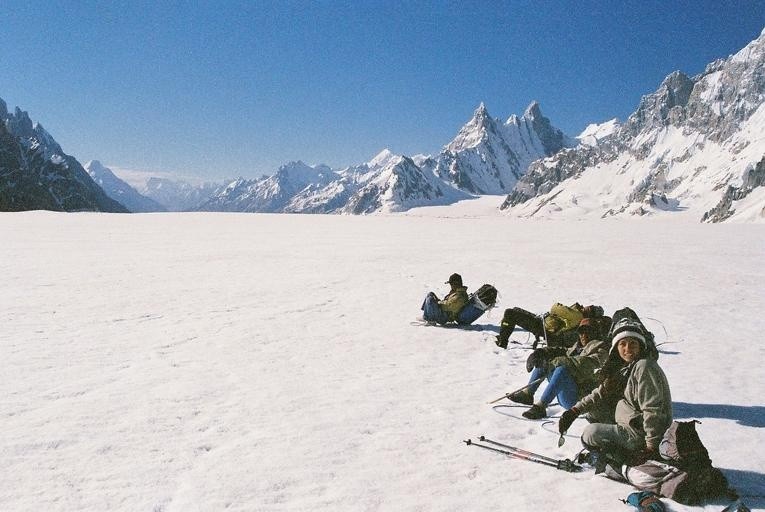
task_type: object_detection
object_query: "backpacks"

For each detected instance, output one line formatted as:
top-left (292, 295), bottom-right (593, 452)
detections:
top-left (455, 284), bottom-right (498, 325)
top-left (614, 416), bottom-right (729, 509)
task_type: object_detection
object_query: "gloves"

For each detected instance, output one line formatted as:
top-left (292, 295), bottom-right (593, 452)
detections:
top-left (526, 348), bottom-right (544, 373)
top-left (557, 409), bottom-right (576, 433)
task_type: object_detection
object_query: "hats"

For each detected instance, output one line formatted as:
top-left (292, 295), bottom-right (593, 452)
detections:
top-left (574, 317), bottom-right (601, 335)
top-left (443, 272), bottom-right (464, 285)
top-left (608, 316), bottom-right (649, 356)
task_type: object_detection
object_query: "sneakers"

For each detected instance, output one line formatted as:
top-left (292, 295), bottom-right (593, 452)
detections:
top-left (522, 405), bottom-right (547, 419)
top-left (506, 389), bottom-right (537, 406)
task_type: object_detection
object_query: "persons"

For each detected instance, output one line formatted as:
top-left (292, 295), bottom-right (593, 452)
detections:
top-left (415, 272), bottom-right (469, 328)
top-left (504, 317), bottom-right (611, 420)
top-left (494, 300), bottom-right (607, 350)
top-left (558, 317), bottom-right (674, 477)
